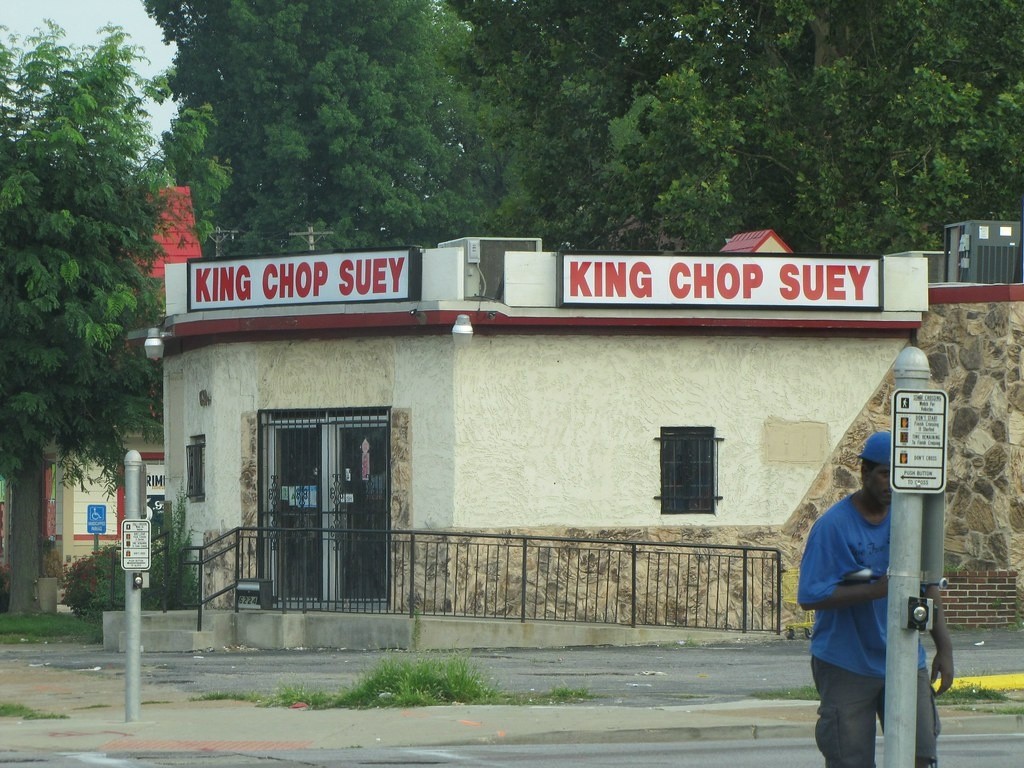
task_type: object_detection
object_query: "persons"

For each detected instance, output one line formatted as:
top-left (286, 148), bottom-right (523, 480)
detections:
top-left (799, 432), bottom-right (954, 768)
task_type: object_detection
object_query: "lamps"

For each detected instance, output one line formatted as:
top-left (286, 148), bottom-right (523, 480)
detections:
top-left (144, 328), bottom-right (172, 358)
top-left (452, 314), bottom-right (474, 343)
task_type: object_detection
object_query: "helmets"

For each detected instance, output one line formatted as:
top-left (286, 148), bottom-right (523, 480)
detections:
top-left (855, 431), bottom-right (893, 464)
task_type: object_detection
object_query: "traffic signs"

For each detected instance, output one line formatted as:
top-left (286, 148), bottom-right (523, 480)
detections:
top-left (121, 519), bottom-right (152, 571)
top-left (891, 388), bottom-right (948, 493)
